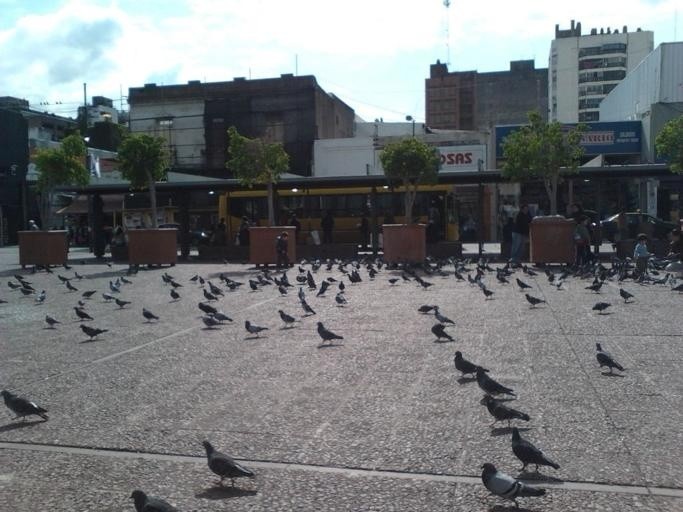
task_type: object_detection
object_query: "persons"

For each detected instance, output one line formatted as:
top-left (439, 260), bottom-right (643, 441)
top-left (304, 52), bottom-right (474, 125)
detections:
top-left (427, 195), bottom-right (445, 237)
top-left (498, 200), bottom-right (534, 268)
top-left (571, 203), bottom-right (683, 281)
top-left (215, 208), bottom-right (396, 269)
top-left (27, 220), bottom-right (40, 231)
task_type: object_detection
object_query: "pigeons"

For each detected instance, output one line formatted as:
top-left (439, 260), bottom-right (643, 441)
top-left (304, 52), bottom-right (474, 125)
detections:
top-left (14, 254), bottom-right (683, 346)
top-left (454, 351), bottom-right (490, 379)
top-left (0, 390), bottom-right (50, 421)
top-left (0, 299), bottom-right (8, 303)
top-left (129, 490), bottom-right (197, 512)
top-left (595, 343), bottom-right (623, 374)
top-left (8, 281), bottom-right (21, 289)
top-left (201, 440), bottom-right (255, 489)
top-left (474, 364), bottom-right (560, 509)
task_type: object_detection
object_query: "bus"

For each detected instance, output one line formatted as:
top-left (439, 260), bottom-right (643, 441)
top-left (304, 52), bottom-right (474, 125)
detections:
top-left (217, 182), bottom-right (460, 245)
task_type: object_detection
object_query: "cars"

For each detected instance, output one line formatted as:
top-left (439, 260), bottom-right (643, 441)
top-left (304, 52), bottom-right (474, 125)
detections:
top-left (591, 208), bottom-right (674, 243)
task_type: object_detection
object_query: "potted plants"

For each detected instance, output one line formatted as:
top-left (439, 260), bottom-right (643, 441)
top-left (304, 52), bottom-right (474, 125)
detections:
top-left (116, 132), bottom-right (180, 266)
top-left (503, 113), bottom-right (589, 267)
top-left (16, 130), bottom-right (89, 270)
top-left (225, 125), bottom-right (298, 268)
top-left (377, 134), bottom-right (442, 264)
